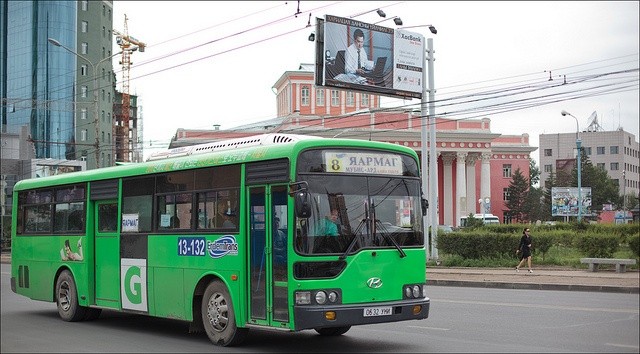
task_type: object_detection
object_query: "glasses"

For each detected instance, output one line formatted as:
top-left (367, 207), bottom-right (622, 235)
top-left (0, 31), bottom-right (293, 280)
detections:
top-left (526, 231), bottom-right (530, 232)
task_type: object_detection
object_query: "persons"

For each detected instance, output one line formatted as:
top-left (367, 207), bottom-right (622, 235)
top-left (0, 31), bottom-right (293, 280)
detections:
top-left (213, 199), bottom-right (234, 227)
top-left (345, 29), bottom-right (377, 76)
top-left (272, 217), bottom-right (287, 253)
top-left (514, 227), bottom-right (535, 274)
top-left (61, 240), bottom-right (84, 261)
top-left (312, 208), bottom-right (340, 236)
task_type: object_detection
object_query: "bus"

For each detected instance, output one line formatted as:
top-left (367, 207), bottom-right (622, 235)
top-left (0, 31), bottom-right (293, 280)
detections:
top-left (460, 213), bottom-right (499, 226)
top-left (10, 132), bottom-right (429, 348)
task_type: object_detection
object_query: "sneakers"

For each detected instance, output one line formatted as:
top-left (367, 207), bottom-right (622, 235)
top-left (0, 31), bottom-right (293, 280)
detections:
top-left (516, 266), bottom-right (519, 273)
top-left (527, 269), bottom-right (533, 273)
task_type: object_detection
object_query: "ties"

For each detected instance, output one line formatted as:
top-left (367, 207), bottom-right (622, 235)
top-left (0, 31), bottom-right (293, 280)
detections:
top-left (357, 50), bottom-right (361, 68)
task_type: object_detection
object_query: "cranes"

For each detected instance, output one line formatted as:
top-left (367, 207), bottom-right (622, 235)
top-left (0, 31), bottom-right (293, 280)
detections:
top-left (113, 13), bottom-right (147, 163)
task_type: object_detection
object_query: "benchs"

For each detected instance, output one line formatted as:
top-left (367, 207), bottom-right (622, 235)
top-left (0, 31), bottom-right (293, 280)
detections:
top-left (580, 257), bottom-right (636, 272)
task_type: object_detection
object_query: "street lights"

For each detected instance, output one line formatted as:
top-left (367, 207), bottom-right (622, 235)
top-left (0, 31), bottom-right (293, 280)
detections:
top-left (561, 110), bottom-right (582, 224)
top-left (47, 37), bottom-right (137, 167)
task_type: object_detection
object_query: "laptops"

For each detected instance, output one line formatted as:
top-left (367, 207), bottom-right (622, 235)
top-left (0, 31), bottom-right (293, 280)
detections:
top-left (361, 57), bottom-right (387, 77)
